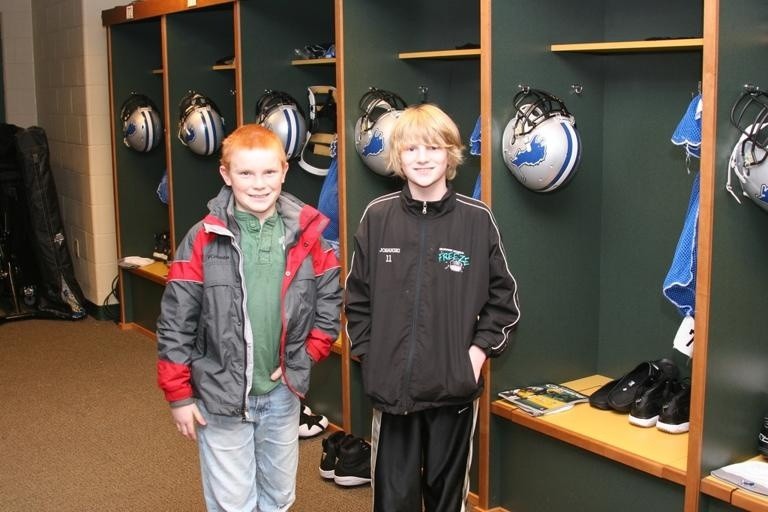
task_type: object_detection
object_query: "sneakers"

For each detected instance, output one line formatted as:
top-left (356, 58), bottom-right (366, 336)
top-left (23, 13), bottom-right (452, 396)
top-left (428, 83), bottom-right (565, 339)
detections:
top-left (589, 359), bottom-right (691, 434)
top-left (152, 231), bottom-right (170, 261)
top-left (320, 431), bottom-right (372, 487)
top-left (287, 39), bottom-right (336, 60)
top-left (298, 405), bottom-right (328, 438)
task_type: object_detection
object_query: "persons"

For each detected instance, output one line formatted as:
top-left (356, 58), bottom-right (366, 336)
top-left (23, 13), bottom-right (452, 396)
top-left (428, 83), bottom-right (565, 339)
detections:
top-left (156, 123), bottom-right (344, 512)
top-left (344, 102), bottom-right (522, 511)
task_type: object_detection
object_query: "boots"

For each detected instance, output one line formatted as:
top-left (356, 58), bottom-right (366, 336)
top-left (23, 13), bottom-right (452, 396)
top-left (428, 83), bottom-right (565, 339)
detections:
top-left (298, 85), bottom-right (337, 177)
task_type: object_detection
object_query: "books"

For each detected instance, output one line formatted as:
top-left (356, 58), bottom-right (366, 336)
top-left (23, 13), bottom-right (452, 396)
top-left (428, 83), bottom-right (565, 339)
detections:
top-left (518, 404), bottom-right (576, 417)
top-left (500, 380), bottom-right (593, 413)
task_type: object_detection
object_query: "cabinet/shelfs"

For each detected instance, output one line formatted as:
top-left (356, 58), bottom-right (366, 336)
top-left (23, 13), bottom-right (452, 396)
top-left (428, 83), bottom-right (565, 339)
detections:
top-left (102, 0), bottom-right (767, 512)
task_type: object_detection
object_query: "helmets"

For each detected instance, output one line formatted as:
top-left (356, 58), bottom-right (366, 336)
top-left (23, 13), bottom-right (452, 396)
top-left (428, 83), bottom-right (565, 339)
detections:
top-left (120, 93), bottom-right (163, 153)
top-left (725, 86), bottom-right (768, 208)
top-left (255, 90), bottom-right (307, 161)
top-left (177, 92), bottom-right (225, 157)
top-left (501, 86), bottom-right (583, 196)
top-left (354, 89), bottom-right (408, 178)
